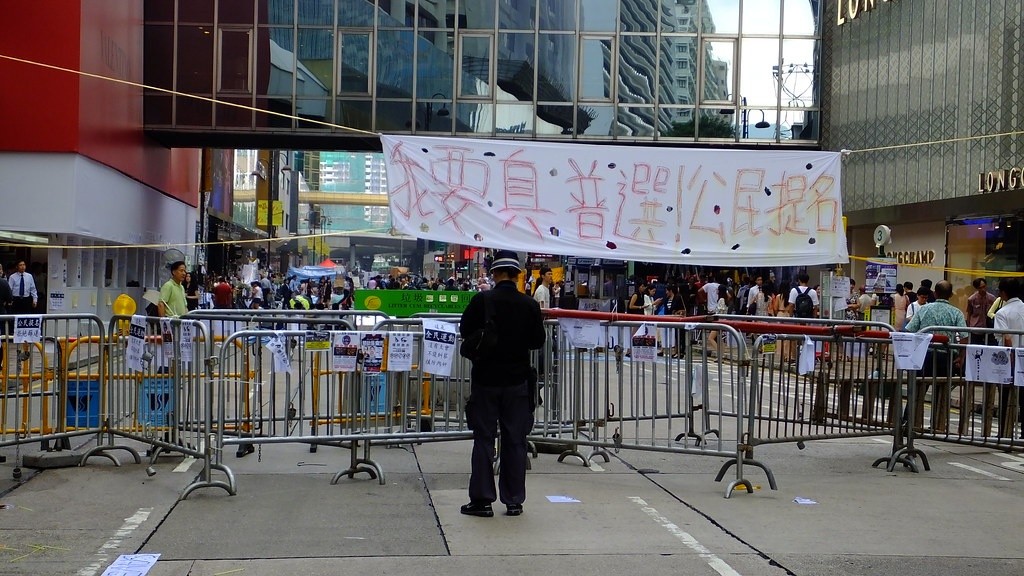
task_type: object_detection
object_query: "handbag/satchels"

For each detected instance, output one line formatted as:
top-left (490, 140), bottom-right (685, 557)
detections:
top-left (856, 311), bottom-right (862, 320)
top-left (749, 302), bottom-right (756, 314)
top-left (905, 295), bottom-right (910, 312)
top-left (627, 292), bottom-right (639, 314)
top-left (902, 303), bottom-right (914, 331)
top-left (460, 290), bottom-right (499, 362)
top-left (986, 297), bottom-right (1003, 328)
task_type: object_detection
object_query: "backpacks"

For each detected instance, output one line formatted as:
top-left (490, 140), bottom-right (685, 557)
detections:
top-left (794, 286), bottom-right (814, 318)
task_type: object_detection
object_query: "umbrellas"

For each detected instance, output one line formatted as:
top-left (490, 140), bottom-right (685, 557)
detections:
top-left (317, 258), bottom-right (336, 267)
top-left (142, 290), bottom-right (161, 307)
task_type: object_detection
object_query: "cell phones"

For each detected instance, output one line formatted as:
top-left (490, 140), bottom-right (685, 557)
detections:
top-left (660, 298), bottom-right (664, 301)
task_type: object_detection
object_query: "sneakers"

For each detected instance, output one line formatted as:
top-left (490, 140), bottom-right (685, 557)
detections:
top-left (460, 500), bottom-right (494, 517)
top-left (506, 503), bottom-right (523, 516)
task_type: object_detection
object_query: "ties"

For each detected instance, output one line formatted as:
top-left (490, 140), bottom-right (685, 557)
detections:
top-left (19, 275), bottom-right (25, 298)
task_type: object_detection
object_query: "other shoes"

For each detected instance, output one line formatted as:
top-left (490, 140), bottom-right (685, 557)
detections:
top-left (657, 351), bottom-right (664, 356)
top-left (672, 353), bottom-right (684, 359)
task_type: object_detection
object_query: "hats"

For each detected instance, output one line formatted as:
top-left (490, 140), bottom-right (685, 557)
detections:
top-left (489, 250), bottom-right (522, 274)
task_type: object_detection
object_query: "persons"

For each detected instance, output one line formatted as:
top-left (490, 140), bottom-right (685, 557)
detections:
top-left (0, 257), bottom-right (38, 370)
top-left (145, 261), bottom-right (1024, 441)
top-left (459, 250), bottom-right (547, 518)
